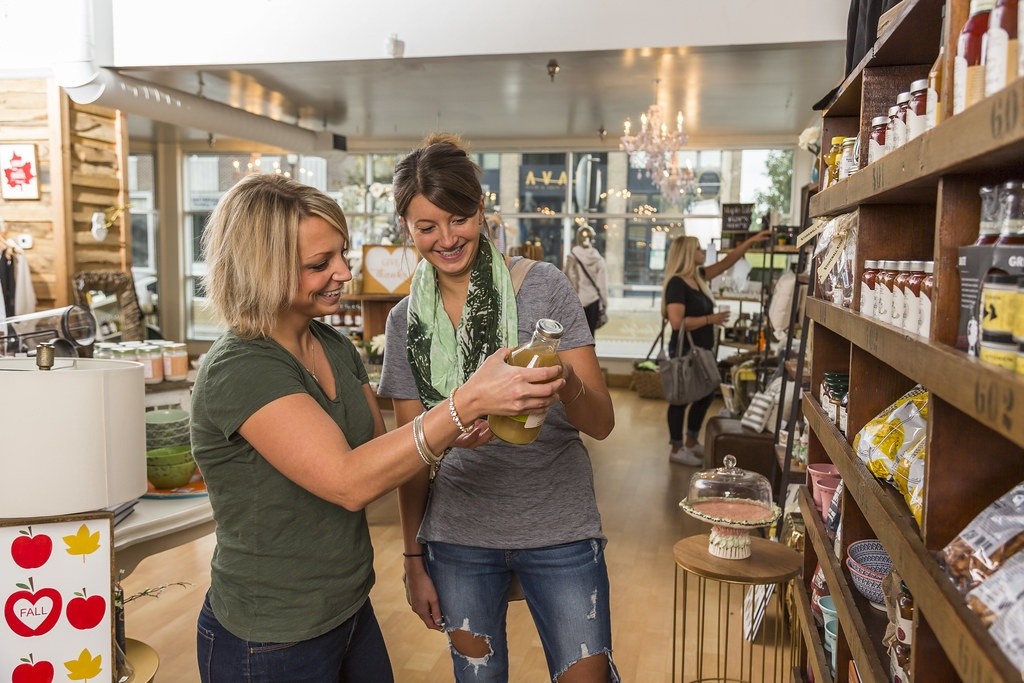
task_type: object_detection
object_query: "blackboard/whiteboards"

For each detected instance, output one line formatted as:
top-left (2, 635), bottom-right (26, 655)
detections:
top-left (721, 203), bottom-right (757, 232)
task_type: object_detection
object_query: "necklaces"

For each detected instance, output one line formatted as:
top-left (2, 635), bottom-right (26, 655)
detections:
top-left (299, 329), bottom-right (318, 382)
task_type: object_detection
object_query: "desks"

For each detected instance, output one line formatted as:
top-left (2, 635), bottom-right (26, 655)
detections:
top-left (672, 533), bottom-right (802, 683)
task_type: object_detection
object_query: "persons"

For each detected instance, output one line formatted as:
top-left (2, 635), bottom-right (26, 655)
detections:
top-left (661, 230), bottom-right (771, 466)
top-left (188, 175), bottom-right (565, 683)
top-left (566, 225), bottom-right (609, 338)
top-left (375, 131), bottom-right (620, 683)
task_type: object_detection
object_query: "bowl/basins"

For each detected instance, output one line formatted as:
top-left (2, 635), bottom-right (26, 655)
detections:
top-left (846, 539), bottom-right (894, 611)
top-left (145, 409), bottom-right (195, 489)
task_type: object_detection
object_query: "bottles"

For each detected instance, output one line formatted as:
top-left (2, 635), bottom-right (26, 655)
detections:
top-left (926, 5), bottom-right (946, 135)
top-left (88, 336), bottom-right (188, 383)
top-left (823, 136), bottom-right (857, 190)
top-left (868, 79), bottom-right (927, 166)
top-left (860, 260), bottom-right (934, 338)
top-left (311, 303), bottom-right (361, 327)
top-left (819, 370), bottom-right (849, 438)
top-left (749, 320), bottom-right (766, 351)
top-left (952, 0), bottom-right (1024, 115)
top-left (488, 319), bottom-right (564, 445)
top-left (969, 181), bottom-right (1024, 374)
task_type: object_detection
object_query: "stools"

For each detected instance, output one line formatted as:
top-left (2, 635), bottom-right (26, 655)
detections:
top-left (704, 416), bottom-right (775, 482)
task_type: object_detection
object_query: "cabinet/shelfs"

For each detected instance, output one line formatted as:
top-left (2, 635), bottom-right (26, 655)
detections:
top-left (712, 238), bottom-right (771, 350)
top-left (790, 0), bottom-right (1024, 683)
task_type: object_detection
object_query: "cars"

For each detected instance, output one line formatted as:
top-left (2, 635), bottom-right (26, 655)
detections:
top-left (134, 262), bottom-right (227, 340)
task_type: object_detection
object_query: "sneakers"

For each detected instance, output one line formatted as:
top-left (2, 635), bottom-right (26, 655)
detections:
top-left (669, 448), bottom-right (703, 466)
top-left (689, 443), bottom-right (704, 457)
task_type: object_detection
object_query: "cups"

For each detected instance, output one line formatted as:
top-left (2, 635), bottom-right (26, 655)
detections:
top-left (806, 463), bottom-right (841, 523)
top-left (818, 595), bottom-right (837, 679)
top-left (718, 304), bottom-right (730, 330)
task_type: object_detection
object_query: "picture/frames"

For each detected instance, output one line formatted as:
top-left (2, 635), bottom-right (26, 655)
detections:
top-left (0, 143), bottom-right (41, 201)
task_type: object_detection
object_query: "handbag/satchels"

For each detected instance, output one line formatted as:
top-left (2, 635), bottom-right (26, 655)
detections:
top-left (594, 309), bottom-right (608, 329)
top-left (657, 319), bottom-right (722, 406)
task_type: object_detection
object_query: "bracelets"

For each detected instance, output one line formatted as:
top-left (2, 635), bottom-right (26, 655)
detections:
top-left (449, 388), bottom-right (475, 433)
top-left (560, 378), bottom-right (585, 410)
top-left (413, 411), bottom-right (444, 484)
top-left (403, 552), bottom-right (423, 557)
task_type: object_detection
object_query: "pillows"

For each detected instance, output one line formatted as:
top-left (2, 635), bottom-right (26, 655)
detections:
top-left (741, 391), bottom-right (775, 432)
top-left (764, 377), bottom-right (795, 434)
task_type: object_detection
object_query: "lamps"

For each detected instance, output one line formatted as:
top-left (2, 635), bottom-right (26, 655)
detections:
top-left (547, 59), bottom-right (560, 82)
top-left (620, 78), bottom-right (689, 186)
